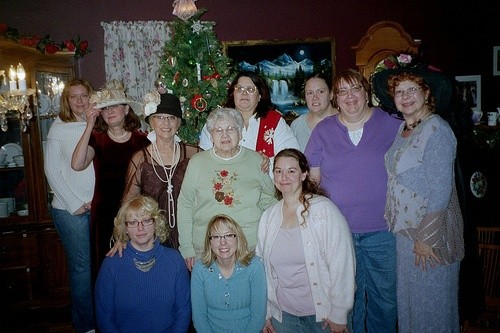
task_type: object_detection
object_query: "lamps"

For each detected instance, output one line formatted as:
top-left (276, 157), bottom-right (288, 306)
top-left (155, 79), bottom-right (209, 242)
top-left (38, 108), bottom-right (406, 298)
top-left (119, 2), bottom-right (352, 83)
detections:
top-left (0, 62), bottom-right (37, 132)
top-left (172, 0), bottom-right (197, 20)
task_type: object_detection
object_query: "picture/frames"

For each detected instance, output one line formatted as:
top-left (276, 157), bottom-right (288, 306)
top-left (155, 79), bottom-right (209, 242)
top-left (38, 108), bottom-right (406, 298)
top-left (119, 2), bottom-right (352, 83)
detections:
top-left (222, 37), bottom-right (335, 119)
top-left (456, 75), bottom-right (482, 112)
top-left (493, 46), bottom-right (500, 76)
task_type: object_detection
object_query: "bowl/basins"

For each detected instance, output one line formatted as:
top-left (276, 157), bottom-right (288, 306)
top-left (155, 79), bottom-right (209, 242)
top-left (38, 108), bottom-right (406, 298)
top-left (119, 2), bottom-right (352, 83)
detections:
top-left (12, 155), bottom-right (25, 167)
top-left (17, 209), bottom-right (29, 216)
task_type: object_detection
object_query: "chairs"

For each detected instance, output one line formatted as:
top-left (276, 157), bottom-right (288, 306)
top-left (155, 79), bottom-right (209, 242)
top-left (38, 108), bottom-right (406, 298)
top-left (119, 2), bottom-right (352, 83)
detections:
top-left (463, 228), bottom-right (500, 333)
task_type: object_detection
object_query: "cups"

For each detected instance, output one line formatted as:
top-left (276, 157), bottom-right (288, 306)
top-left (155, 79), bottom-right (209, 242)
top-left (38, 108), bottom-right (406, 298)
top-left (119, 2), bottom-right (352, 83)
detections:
top-left (472, 110), bottom-right (483, 125)
top-left (0, 198), bottom-right (16, 217)
top-left (487, 112), bottom-right (498, 126)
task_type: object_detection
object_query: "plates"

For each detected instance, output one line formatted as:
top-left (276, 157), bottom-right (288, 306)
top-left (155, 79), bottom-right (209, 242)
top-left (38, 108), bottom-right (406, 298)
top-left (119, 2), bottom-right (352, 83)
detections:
top-left (0, 143), bottom-right (22, 164)
top-left (470, 172), bottom-right (487, 198)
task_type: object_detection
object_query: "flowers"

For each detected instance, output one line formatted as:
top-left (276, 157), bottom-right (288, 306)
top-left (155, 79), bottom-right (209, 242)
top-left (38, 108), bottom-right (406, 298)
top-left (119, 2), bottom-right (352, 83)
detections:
top-left (0, 24), bottom-right (92, 57)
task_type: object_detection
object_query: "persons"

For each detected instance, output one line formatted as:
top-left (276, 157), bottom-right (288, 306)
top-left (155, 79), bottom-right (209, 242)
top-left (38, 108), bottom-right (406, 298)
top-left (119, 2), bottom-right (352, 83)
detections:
top-left (199, 69), bottom-right (299, 182)
top-left (302, 70), bottom-right (403, 333)
top-left (255, 148), bottom-right (356, 333)
top-left (290, 72), bottom-right (341, 154)
top-left (96, 194), bottom-right (191, 333)
top-left (71, 81), bottom-right (152, 333)
top-left (107, 92), bottom-right (204, 257)
top-left (44, 81), bottom-right (95, 333)
top-left (177, 107), bottom-right (279, 272)
top-left (191, 214), bottom-right (266, 333)
top-left (369, 51), bottom-right (465, 333)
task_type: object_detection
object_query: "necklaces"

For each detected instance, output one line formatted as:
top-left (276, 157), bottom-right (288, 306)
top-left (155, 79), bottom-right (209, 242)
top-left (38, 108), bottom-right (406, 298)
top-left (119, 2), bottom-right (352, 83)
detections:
top-left (126, 246), bottom-right (164, 273)
top-left (403, 111), bottom-right (431, 131)
top-left (212, 146), bottom-right (242, 161)
top-left (108, 130), bottom-right (127, 141)
top-left (150, 139), bottom-right (180, 228)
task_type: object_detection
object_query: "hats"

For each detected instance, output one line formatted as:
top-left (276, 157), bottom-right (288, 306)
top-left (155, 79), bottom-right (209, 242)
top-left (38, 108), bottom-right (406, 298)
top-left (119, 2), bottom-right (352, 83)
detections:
top-left (143, 91), bottom-right (186, 127)
top-left (89, 88), bottom-right (142, 115)
top-left (372, 54), bottom-right (458, 118)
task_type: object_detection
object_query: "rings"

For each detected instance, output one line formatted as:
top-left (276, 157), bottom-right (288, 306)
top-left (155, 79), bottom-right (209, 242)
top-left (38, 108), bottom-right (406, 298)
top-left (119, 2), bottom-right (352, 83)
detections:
top-left (427, 256), bottom-right (431, 260)
top-left (90, 111), bottom-right (93, 114)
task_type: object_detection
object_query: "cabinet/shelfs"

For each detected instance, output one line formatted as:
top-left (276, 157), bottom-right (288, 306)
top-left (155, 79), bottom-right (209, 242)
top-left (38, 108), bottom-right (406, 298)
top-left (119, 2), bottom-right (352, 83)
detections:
top-left (0, 39), bottom-right (76, 333)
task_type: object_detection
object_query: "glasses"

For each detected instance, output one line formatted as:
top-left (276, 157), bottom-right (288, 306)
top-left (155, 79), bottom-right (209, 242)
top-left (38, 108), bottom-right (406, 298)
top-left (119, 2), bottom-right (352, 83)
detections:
top-left (213, 126), bottom-right (238, 134)
top-left (209, 233), bottom-right (237, 241)
top-left (233, 85), bottom-right (258, 95)
top-left (336, 84), bottom-right (364, 96)
top-left (125, 218), bottom-right (155, 228)
top-left (394, 86), bottom-right (424, 97)
